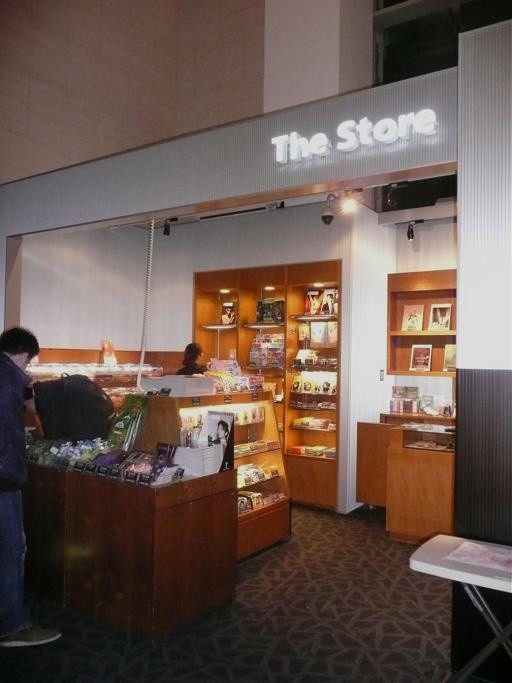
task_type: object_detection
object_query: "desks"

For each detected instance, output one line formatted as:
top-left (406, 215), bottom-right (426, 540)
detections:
top-left (408, 532), bottom-right (512, 682)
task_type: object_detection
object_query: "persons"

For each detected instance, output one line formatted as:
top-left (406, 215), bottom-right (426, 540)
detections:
top-left (207, 419), bottom-right (229, 453)
top-left (220, 304), bottom-right (237, 324)
top-left (0, 327), bottom-right (62, 648)
top-left (176, 343), bottom-right (207, 376)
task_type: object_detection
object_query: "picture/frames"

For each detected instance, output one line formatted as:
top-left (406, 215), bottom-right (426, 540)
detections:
top-left (428, 303), bottom-right (452, 330)
top-left (409, 343), bottom-right (432, 372)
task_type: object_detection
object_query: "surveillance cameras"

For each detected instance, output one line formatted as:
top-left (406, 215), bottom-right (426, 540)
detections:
top-left (385, 188), bottom-right (401, 210)
top-left (321, 207), bottom-right (337, 225)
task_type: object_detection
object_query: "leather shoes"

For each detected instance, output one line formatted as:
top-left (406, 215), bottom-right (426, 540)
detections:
top-left (0, 623), bottom-right (63, 649)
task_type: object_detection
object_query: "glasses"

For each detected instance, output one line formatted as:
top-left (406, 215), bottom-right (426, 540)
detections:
top-left (25, 357), bottom-right (32, 369)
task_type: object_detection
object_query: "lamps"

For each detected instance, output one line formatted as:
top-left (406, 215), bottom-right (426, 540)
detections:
top-left (319, 193), bottom-right (340, 224)
top-left (161, 216), bottom-right (179, 237)
top-left (407, 219), bottom-right (425, 240)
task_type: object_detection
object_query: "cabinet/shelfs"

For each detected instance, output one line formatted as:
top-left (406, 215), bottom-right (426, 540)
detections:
top-left (355, 411), bottom-right (455, 546)
top-left (386, 287), bottom-right (458, 377)
top-left (123, 388), bottom-right (293, 563)
top-left (193, 273), bottom-right (343, 510)
top-left (23, 360), bottom-right (164, 412)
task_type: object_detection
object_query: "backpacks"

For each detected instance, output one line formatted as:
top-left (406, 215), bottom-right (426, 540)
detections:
top-left (32, 373), bottom-right (116, 441)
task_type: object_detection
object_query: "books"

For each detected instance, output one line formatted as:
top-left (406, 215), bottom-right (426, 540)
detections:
top-left (248, 333), bottom-right (286, 368)
top-left (392, 303), bottom-right (456, 417)
top-left (285, 287), bottom-right (339, 459)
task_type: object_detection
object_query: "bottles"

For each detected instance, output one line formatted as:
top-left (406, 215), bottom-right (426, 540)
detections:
top-left (390, 398), bottom-right (422, 414)
